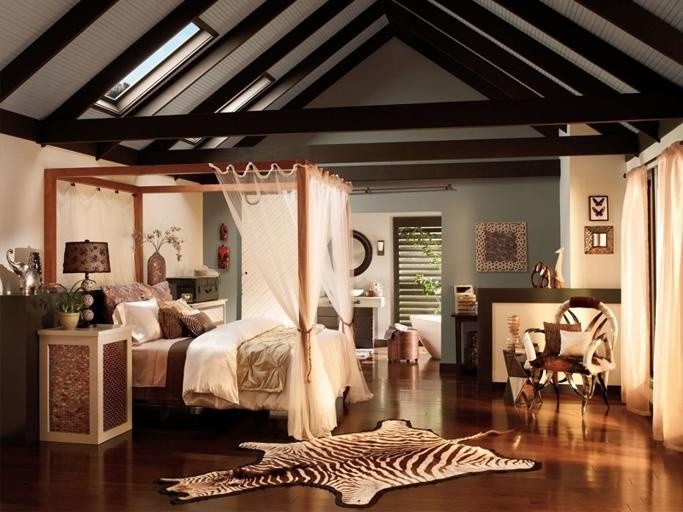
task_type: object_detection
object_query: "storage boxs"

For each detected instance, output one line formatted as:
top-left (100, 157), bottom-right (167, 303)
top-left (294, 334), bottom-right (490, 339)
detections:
top-left (166, 274), bottom-right (218, 305)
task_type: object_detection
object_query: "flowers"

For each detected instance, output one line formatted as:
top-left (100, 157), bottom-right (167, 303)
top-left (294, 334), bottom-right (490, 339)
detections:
top-left (128, 222), bottom-right (184, 262)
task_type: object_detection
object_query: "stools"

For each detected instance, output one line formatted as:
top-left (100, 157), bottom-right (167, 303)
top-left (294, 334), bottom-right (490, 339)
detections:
top-left (383, 322), bottom-right (418, 364)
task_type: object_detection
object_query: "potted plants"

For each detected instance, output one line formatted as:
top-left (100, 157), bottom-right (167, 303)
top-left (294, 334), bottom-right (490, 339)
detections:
top-left (38, 277), bottom-right (96, 330)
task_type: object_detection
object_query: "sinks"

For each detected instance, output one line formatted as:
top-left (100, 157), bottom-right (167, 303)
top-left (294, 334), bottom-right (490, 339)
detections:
top-left (353, 289), bottom-right (366, 297)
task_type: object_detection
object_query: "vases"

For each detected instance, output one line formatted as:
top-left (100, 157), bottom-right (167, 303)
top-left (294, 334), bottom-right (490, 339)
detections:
top-left (145, 251), bottom-right (167, 285)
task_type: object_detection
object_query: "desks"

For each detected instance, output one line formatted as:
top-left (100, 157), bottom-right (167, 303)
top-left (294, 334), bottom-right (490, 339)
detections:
top-left (502, 344), bottom-right (542, 406)
top-left (449, 311), bottom-right (478, 378)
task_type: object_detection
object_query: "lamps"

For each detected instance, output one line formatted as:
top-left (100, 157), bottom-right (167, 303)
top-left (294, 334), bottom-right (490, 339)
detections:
top-left (375, 239), bottom-right (386, 257)
top-left (61, 237), bottom-right (111, 330)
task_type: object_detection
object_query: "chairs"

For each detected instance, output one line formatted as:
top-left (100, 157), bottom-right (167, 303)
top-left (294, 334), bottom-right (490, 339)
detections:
top-left (522, 295), bottom-right (620, 415)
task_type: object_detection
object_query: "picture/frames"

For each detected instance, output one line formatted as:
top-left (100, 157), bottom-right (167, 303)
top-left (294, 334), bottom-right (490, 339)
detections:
top-left (586, 194), bottom-right (610, 223)
top-left (473, 218), bottom-right (529, 274)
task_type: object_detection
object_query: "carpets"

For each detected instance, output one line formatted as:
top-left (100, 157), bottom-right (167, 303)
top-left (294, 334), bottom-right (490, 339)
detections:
top-left (152, 415), bottom-right (545, 509)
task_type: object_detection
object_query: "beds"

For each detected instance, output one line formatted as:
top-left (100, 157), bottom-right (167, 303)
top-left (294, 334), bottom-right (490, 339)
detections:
top-left (40, 156), bottom-right (376, 443)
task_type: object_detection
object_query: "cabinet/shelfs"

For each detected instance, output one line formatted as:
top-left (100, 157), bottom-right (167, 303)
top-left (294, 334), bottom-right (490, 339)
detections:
top-left (315, 295), bottom-right (386, 350)
top-left (36, 323), bottom-right (135, 446)
top-left (188, 297), bottom-right (230, 329)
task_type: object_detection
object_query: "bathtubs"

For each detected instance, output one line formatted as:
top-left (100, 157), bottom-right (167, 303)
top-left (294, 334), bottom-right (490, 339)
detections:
top-left (409, 313), bottom-right (443, 360)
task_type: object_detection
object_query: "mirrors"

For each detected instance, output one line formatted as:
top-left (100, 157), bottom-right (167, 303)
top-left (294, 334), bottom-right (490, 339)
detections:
top-left (326, 229), bottom-right (373, 278)
top-left (582, 225), bottom-right (615, 256)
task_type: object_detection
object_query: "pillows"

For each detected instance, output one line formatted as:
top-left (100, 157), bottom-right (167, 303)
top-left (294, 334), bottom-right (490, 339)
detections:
top-left (556, 328), bottom-right (596, 357)
top-left (108, 297), bottom-right (165, 347)
top-left (157, 306), bottom-right (190, 340)
top-left (163, 297), bottom-right (199, 314)
top-left (177, 310), bottom-right (215, 336)
top-left (137, 280), bottom-right (174, 303)
top-left (542, 321), bottom-right (582, 357)
top-left (99, 281), bottom-right (146, 324)
